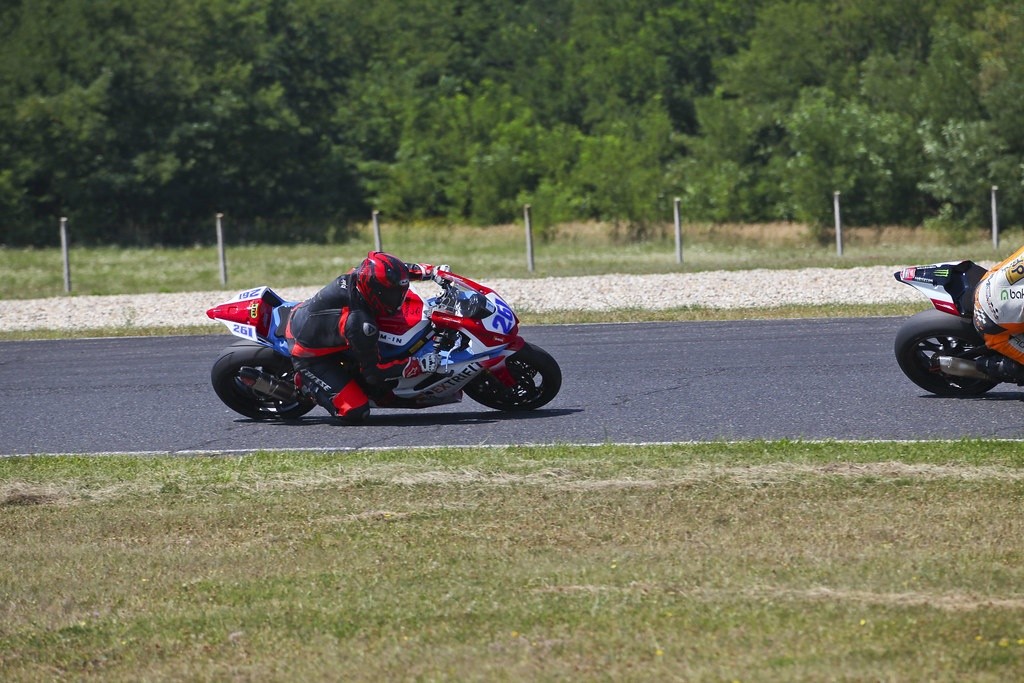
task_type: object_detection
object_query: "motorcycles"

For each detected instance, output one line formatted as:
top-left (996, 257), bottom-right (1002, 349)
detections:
top-left (204, 268), bottom-right (563, 423)
top-left (891, 260), bottom-right (1024, 396)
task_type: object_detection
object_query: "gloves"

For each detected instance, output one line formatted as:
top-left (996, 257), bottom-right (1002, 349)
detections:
top-left (433, 265), bottom-right (450, 286)
top-left (419, 354), bottom-right (441, 374)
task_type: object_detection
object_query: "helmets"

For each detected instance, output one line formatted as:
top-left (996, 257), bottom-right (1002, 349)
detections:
top-left (356, 251), bottom-right (410, 318)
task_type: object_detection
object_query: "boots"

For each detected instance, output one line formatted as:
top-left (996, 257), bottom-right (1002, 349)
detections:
top-left (294, 371), bottom-right (317, 404)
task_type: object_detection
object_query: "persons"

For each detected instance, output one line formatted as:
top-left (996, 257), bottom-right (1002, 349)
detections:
top-left (972, 244), bottom-right (1024, 368)
top-left (284, 249), bottom-right (452, 424)
